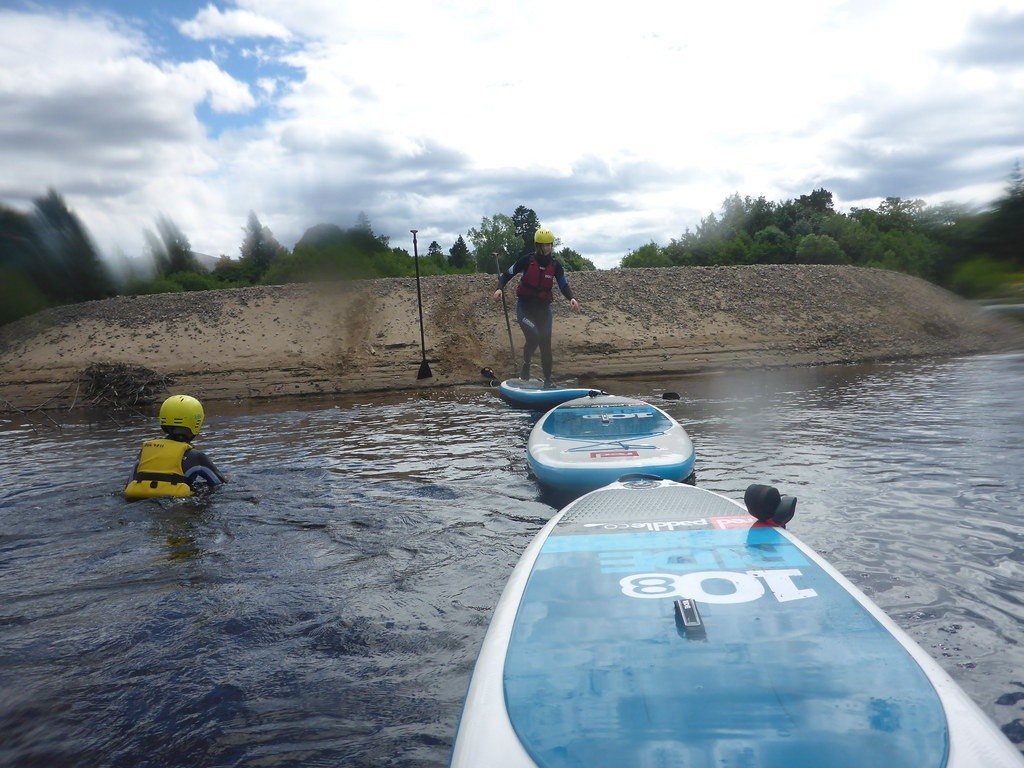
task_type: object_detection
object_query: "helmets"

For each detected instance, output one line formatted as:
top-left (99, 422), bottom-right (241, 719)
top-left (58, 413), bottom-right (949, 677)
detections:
top-left (533, 229), bottom-right (554, 244)
top-left (159, 394), bottom-right (205, 436)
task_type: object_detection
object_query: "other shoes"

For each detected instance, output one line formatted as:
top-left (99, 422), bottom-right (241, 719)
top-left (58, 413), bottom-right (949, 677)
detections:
top-left (544, 379), bottom-right (557, 389)
top-left (520, 363), bottom-right (530, 381)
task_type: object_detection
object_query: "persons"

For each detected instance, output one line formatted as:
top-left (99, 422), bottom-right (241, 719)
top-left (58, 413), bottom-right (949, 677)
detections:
top-left (128, 394), bottom-right (226, 499)
top-left (494, 230), bottom-right (580, 389)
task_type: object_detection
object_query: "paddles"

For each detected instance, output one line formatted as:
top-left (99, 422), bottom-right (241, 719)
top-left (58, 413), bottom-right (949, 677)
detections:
top-left (491, 252), bottom-right (519, 377)
top-left (410, 230), bottom-right (433, 380)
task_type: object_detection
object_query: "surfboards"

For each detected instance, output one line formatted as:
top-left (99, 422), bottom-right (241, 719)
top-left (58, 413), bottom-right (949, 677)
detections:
top-left (448, 472), bottom-right (1024, 768)
top-left (500, 374), bottom-right (610, 409)
top-left (526, 394), bottom-right (696, 493)
